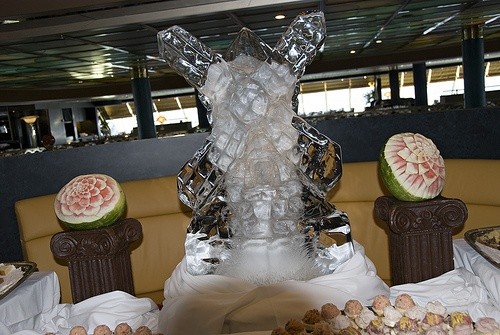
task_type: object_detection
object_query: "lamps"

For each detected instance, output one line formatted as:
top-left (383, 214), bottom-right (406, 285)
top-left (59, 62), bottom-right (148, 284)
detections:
top-left (156, 116), bottom-right (167, 123)
top-left (22, 115), bottom-right (39, 123)
